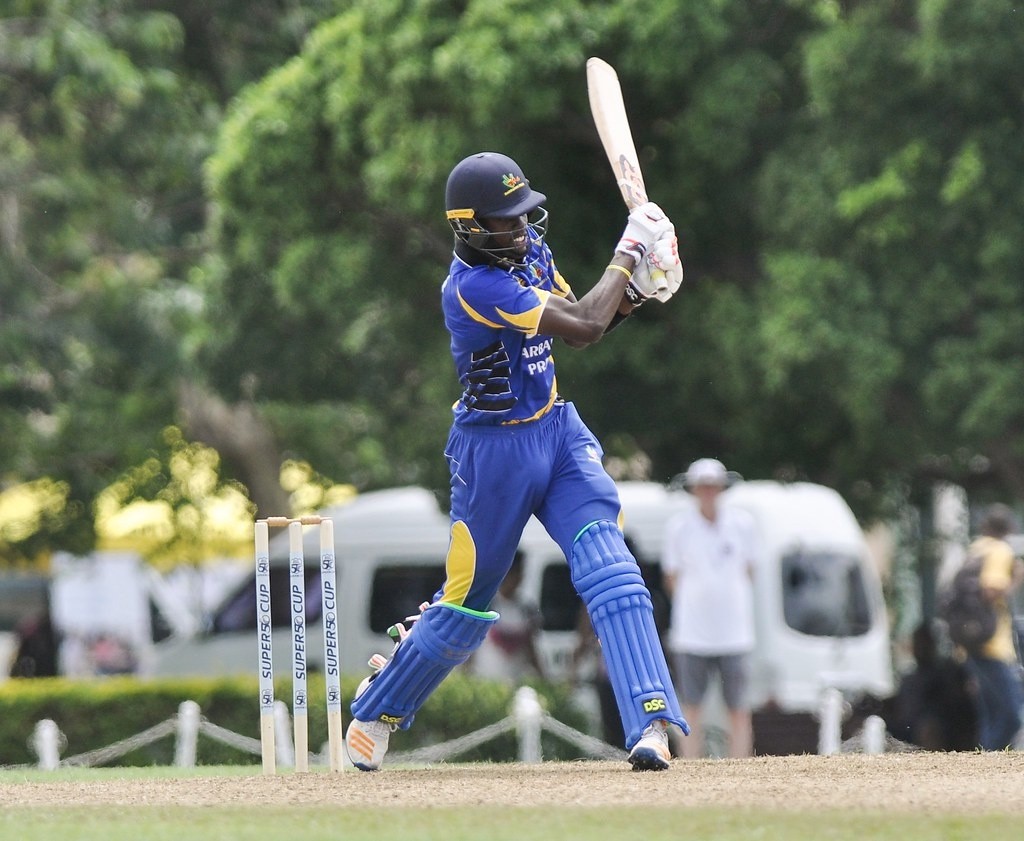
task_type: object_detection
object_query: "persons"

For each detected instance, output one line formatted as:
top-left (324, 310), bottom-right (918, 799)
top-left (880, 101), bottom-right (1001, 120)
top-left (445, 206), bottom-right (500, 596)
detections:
top-left (346, 151), bottom-right (691, 773)
top-left (951, 504), bottom-right (1023, 752)
top-left (460, 459), bottom-right (765, 760)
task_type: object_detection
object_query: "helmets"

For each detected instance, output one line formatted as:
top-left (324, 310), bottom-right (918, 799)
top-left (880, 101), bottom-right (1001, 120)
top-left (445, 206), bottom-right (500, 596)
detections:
top-left (445, 151), bottom-right (547, 250)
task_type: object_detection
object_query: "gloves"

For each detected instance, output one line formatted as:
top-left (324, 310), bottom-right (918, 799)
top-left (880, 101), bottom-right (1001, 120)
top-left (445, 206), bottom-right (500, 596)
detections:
top-left (614, 200), bottom-right (670, 267)
top-left (623, 233), bottom-right (684, 308)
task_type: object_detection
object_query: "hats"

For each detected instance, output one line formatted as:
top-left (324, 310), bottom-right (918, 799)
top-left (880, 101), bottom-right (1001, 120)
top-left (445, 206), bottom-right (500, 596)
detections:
top-left (684, 457), bottom-right (728, 487)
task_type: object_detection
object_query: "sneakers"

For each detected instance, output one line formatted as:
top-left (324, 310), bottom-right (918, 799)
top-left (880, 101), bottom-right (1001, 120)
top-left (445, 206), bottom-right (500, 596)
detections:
top-left (628, 721), bottom-right (672, 770)
top-left (345, 672), bottom-right (398, 771)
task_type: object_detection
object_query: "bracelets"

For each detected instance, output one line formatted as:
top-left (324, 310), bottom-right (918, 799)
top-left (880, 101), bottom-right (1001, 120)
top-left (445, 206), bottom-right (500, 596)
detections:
top-left (603, 309), bottom-right (631, 337)
top-left (605, 264), bottom-right (631, 279)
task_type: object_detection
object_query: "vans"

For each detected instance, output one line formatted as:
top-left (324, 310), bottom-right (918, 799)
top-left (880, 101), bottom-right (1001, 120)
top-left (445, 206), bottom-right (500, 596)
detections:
top-left (124, 476), bottom-right (898, 755)
